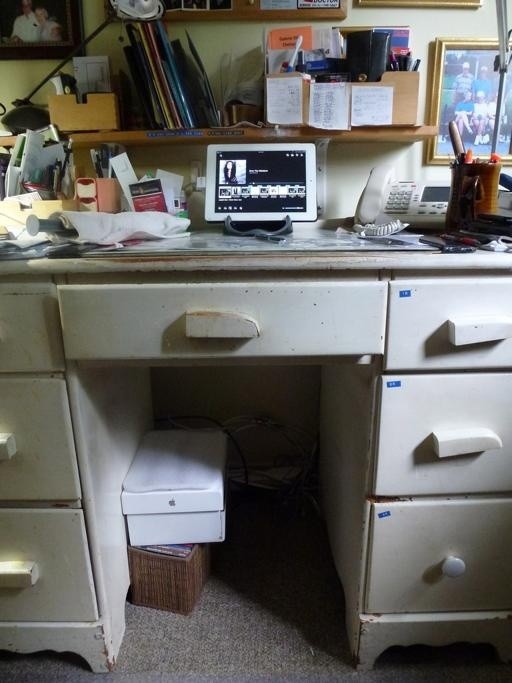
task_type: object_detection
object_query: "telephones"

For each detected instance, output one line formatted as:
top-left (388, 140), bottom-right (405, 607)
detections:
top-left (353, 167), bottom-right (452, 230)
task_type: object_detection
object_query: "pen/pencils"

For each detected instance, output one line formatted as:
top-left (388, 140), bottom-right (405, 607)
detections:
top-left (439, 234), bottom-right (481, 247)
top-left (90, 144), bottom-right (119, 178)
top-left (388, 51), bottom-right (420, 71)
top-left (449, 120), bottom-right (501, 165)
top-left (256, 234), bottom-right (288, 242)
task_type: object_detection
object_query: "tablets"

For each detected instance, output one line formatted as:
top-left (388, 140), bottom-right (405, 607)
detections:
top-left (204, 142), bottom-right (318, 225)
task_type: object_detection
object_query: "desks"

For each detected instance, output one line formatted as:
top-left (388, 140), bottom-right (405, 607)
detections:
top-left (0, 231), bottom-right (512, 673)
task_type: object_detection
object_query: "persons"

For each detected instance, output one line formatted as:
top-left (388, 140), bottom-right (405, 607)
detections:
top-left (8, 1), bottom-right (64, 42)
top-left (223, 161), bottom-right (238, 184)
top-left (451, 61), bottom-right (511, 144)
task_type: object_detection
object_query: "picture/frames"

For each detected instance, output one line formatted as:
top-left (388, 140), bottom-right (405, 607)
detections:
top-left (355, 0), bottom-right (483, 7)
top-left (0, 0), bottom-right (85, 60)
top-left (425, 38), bottom-right (512, 164)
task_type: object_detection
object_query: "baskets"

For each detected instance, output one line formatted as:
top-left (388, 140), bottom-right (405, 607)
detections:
top-left (127, 543), bottom-right (214, 616)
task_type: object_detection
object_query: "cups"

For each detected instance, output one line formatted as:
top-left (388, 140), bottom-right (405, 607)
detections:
top-left (443, 160), bottom-right (503, 229)
top-left (97, 177), bottom-right (124, 212)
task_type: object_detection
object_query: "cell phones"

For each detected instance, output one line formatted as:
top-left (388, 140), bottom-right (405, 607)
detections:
top-left (420, 235), bottom-right (476, 252)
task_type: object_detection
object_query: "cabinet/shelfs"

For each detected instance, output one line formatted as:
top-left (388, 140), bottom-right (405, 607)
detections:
top-left (163, 0), bottom-right (349, 20)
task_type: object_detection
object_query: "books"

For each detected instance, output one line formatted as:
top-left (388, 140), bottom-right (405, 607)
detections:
top-left (262, 26), bottom-right (411, 131)
top-left (73, 55), bottom-right (114, 104)
top-left (134, 17), bottom-right (195, 130)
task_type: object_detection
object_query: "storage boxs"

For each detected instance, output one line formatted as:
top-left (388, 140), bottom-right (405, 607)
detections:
top-left (123, 429), bottom-right (227, 546)
top-left (47, 92), bottom-right (120, 133)
